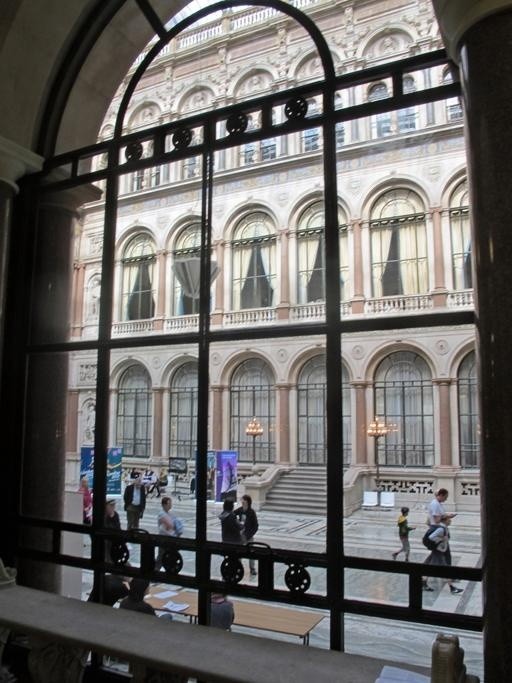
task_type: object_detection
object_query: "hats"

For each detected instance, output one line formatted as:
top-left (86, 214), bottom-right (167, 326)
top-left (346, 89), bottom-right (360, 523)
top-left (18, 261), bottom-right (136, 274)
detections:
top-left (105, 496), bottom-right (120, 504)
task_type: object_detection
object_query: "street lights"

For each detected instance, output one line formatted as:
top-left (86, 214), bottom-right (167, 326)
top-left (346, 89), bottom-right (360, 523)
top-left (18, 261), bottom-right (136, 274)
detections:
top-left (245, 417), bottom-right (264, 467)
top-left (367, 416), bottom-right (390, 507)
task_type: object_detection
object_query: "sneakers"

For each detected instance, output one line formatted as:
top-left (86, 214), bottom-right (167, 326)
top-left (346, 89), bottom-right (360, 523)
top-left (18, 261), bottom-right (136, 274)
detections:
top-left (423, 586), bottom-right (464, 594)
top-left (251, 569), bottom-right (258, 576)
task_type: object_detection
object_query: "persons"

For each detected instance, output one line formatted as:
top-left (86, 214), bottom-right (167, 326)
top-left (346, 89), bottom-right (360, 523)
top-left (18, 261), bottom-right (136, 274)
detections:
top-left (85, 568), bottom-right (129, 607)
top-left (220, 495), bottom-right (245, 546)
top-left (208, 591), bottom-right (236, 631)
top-left (140, 464), bottom-right (158, 496)
top-left (117, 577), bottom-right (173, 619)
top-left (427, 488), bottom-right (457, 526)
top-left (189, 469), bottom-right (194, 493)
top-left (391, 506), bottom-right (416, 563)
top-left (76, 469), bottom-right (90, 522)
top-left (234, 489), bottom-right (260, 575)
top-left (156, 493), bottom-right (183, 571)
top-left (124, 470), bottom-right (151, 529)
top-left (123, 466), bottom-right (140, 485)
top-left (149, 467), bottom-right (170, 498)
top-left (421, 510), bottom-right (464, 595)
top-left (103, 498), bottom-right (123, 566)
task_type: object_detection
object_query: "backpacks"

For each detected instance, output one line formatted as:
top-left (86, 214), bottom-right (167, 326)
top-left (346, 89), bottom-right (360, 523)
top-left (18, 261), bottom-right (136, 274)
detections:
top-left (421, 523), bottom-right (446, 550)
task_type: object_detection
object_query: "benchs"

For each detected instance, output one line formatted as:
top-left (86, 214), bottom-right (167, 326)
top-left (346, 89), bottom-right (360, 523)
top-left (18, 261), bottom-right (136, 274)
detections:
top-left (125, 480), bottom-right (168, 496)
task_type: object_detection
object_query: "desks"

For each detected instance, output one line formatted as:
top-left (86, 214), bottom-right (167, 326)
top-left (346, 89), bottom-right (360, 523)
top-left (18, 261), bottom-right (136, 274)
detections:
top-left (85, 577), bottom-right (326, 644)
